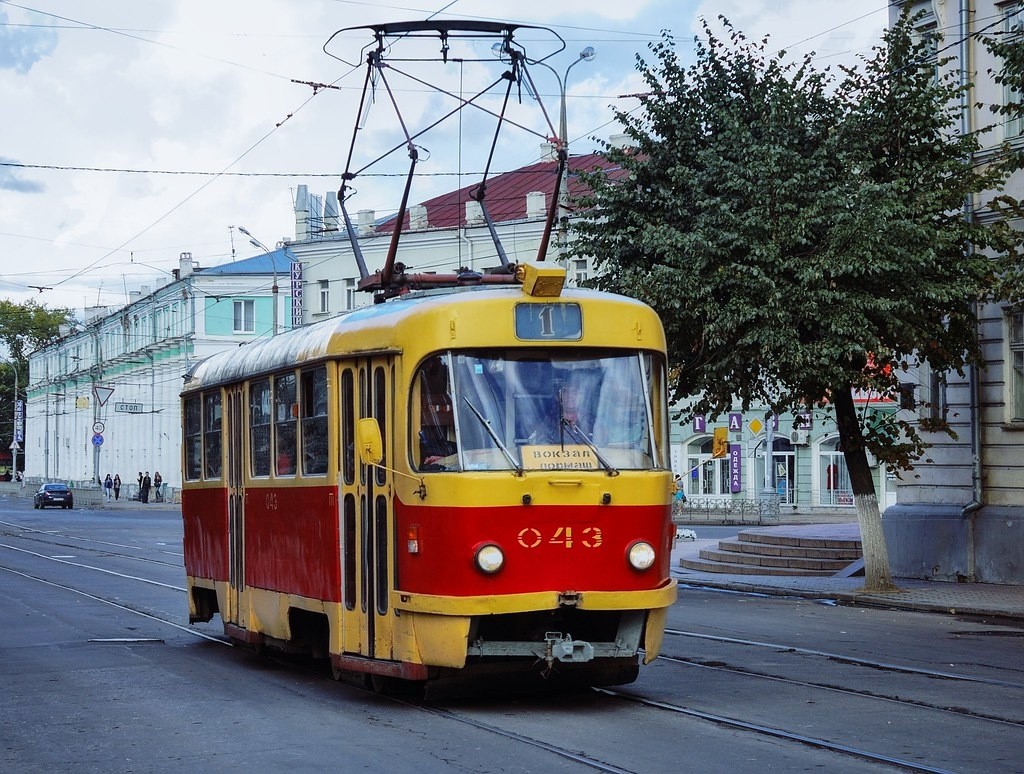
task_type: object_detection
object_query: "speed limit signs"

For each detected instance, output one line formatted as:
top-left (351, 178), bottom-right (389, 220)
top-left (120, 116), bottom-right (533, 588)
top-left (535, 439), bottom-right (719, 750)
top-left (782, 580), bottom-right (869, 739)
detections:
top-left (93, 422), bottom-right (105, 434)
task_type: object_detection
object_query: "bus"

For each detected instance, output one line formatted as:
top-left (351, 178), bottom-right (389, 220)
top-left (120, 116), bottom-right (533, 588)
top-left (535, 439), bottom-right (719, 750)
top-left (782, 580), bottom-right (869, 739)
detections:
top-left (179, 262), bottom-right (679, 708)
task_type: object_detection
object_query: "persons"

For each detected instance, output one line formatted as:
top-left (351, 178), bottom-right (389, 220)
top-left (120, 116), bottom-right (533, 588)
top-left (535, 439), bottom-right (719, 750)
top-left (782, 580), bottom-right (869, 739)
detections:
top-left (141, 471), bottom-right (151, 504)
top-left (154, 472), bottom-right (162, 500)
top-left (104, 474), bottom-right (113, 503)
top-left (113, 474), bottom-right (121, 500)
top-left (534, 377), bottom-right (609, 446)
top-left (137, 472), bottom-right (143, 496)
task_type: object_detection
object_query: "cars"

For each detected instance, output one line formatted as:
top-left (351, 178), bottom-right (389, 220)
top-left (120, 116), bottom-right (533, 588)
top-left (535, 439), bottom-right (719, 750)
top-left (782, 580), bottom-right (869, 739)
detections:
top-left (33, 482), bottom-right (73, 511)
